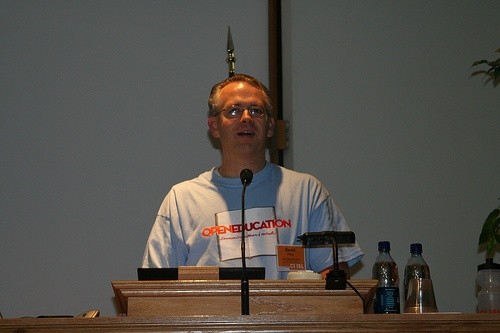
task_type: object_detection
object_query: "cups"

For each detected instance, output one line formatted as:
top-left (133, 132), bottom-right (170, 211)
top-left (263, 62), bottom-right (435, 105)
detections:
top-left (403, 277), bottom-right (438, 313)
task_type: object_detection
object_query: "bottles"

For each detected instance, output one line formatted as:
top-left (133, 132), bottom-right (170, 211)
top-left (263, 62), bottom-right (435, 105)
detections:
top-left (372, 241), bottom-right (400, 314)
top-left (476, 258), bottom-right (500, 313)
top-left (404, 243), bottom-right (430, 299)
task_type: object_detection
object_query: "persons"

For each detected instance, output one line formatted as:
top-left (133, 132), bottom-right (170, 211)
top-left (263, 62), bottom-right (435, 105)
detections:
top-left (142, 73), bottom-right (365, 280)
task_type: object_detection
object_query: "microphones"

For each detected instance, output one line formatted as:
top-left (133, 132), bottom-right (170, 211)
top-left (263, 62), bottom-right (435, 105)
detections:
top-left (240, 169), bottom-right (253, 315)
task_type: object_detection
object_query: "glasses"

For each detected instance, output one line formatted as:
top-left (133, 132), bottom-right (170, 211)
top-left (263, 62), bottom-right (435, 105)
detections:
top-left (213, 105), bottom-right (269, 118)
